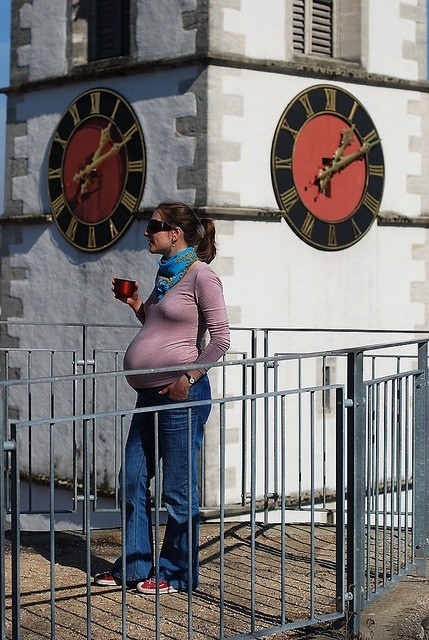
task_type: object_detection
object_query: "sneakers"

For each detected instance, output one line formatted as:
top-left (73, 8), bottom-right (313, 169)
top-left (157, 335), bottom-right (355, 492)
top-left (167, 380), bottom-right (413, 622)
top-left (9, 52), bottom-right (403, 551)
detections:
top-left (136, 577), bottom-right (180, 595)
top-left (93, 570), bottom-right (121, 586)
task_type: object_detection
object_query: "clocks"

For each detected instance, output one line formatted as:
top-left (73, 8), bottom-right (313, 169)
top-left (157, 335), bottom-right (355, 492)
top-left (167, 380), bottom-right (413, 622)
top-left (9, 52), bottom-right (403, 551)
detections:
top-left (46, 86), bottom-right (147, 254)
top-left (270, 84), bottom-right (385, 251)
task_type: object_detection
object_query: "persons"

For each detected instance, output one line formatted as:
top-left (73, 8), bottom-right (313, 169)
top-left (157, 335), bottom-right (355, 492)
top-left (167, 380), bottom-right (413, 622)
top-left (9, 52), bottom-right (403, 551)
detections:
top-left (93, 201), bottom-right (231, 595)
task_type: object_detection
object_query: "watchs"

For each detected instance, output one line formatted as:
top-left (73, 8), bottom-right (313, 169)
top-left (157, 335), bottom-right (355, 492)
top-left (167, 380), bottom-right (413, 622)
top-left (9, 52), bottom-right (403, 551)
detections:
top-left (184, 372), bottom-right (196, 385)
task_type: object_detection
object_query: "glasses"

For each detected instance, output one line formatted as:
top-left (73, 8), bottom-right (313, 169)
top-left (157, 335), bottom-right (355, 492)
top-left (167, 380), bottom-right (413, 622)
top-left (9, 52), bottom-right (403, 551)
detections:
top-left (146, 219), bottom-right (176, 235)
